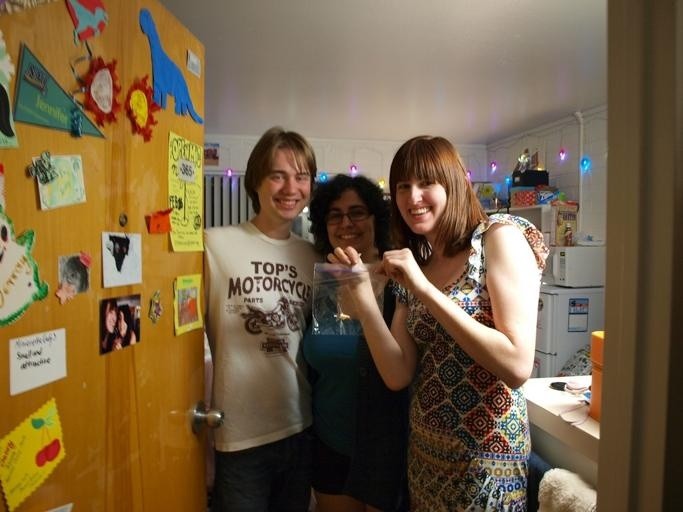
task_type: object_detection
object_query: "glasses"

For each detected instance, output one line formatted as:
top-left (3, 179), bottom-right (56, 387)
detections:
top-left (324, 209), bottom-right (367, 224)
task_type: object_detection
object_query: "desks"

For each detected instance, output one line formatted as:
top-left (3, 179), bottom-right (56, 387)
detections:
top-left (516, 374), bottom-right (599, 467)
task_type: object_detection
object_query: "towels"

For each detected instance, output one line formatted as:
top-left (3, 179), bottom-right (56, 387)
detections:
top-left (535, 467), bottom-right (598, 511)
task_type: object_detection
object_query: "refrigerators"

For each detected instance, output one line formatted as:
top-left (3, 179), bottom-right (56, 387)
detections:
top-left (530, 281), bottom-right (605, 380)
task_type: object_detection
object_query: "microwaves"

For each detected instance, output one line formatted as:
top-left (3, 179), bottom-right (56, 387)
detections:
top-left (532, 238), bottom-right (605, 287)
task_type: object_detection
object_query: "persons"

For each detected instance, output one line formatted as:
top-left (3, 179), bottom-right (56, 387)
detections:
top-left (323, 135), bottom-right (551, 512)
top-left (301, 173), bottom-right (410, 512)
top-left (202, 126), bottom-right (327, 512)
top-left (111, 304), bottom-right (137, 349)
top-left (101, 298), bottom-right (119, 354)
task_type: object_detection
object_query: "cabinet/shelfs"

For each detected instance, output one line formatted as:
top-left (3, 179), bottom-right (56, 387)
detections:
top-left (483, 204), bottom-right (551, 244)
